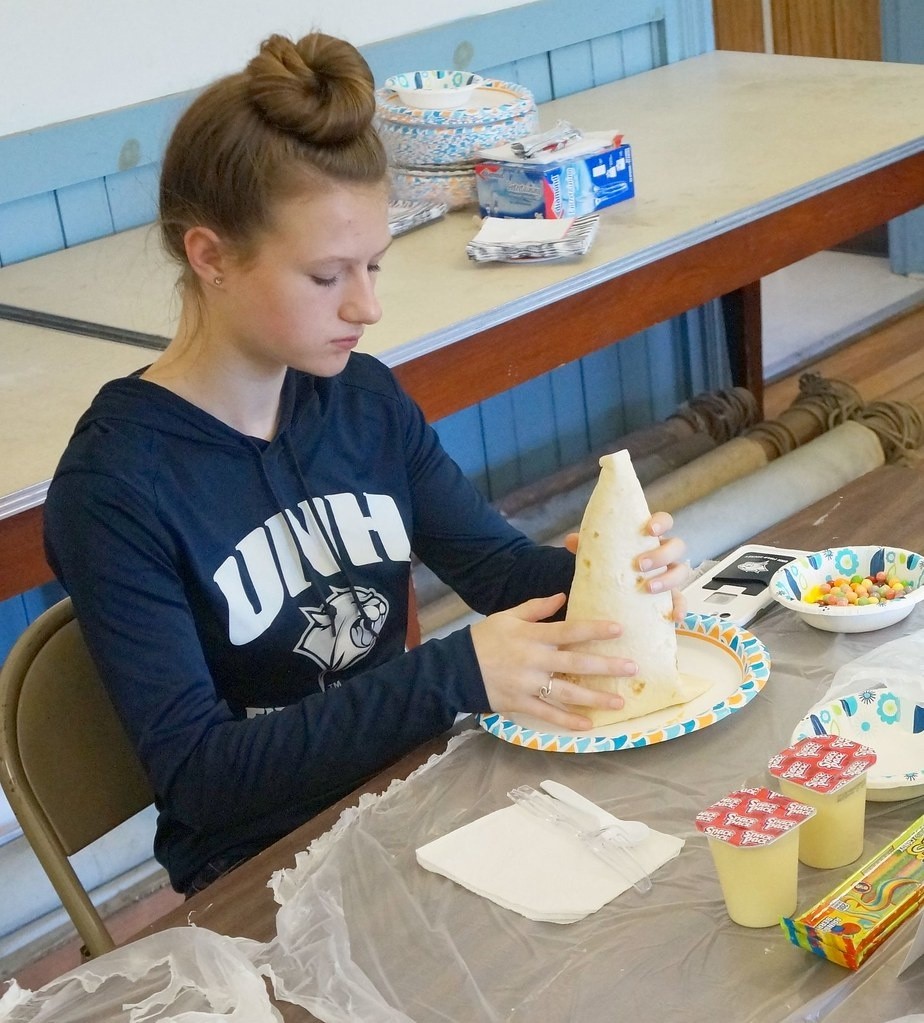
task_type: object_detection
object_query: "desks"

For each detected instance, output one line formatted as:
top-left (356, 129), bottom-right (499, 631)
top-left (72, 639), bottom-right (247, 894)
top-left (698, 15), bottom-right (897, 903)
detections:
top-left (0, 50), bottom-right (924, 600)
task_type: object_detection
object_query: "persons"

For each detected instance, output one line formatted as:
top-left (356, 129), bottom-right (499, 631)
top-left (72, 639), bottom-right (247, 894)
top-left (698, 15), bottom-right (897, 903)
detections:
top-left (42, 34), bottom-right (690, 902)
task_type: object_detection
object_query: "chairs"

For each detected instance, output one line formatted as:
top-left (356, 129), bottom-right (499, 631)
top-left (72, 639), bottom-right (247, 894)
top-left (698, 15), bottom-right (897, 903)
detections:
top-left (0, 599), bottom-right (181, 963)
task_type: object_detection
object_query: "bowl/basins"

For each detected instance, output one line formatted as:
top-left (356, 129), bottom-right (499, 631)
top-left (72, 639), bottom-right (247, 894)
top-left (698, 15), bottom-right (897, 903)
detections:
top-left (768, 545), bottom-right (924, 633)
top-left (792, 688), bottom-right (924, 802)
top-left (385, 70), bottom-right (483, 110)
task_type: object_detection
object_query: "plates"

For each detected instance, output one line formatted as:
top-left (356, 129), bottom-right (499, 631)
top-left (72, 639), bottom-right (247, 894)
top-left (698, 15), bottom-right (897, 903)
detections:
top-left (374, 80), bottom-right (539, 204)
top-left (474, 611), bottom-right (770, 753)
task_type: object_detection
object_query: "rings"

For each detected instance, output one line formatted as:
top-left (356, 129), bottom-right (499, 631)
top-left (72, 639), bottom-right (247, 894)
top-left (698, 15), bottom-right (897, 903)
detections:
top-left (539, 679), bottom-right (552, 700)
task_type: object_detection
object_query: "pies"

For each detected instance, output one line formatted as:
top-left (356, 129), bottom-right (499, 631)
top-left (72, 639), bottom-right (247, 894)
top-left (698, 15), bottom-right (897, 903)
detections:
top-left (551, 449), bottom-right (713, 727)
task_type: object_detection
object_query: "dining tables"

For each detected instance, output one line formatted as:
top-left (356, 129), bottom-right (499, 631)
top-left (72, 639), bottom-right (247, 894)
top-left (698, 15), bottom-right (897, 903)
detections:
top-left (0, 445), bottom-right (924, 1023)
